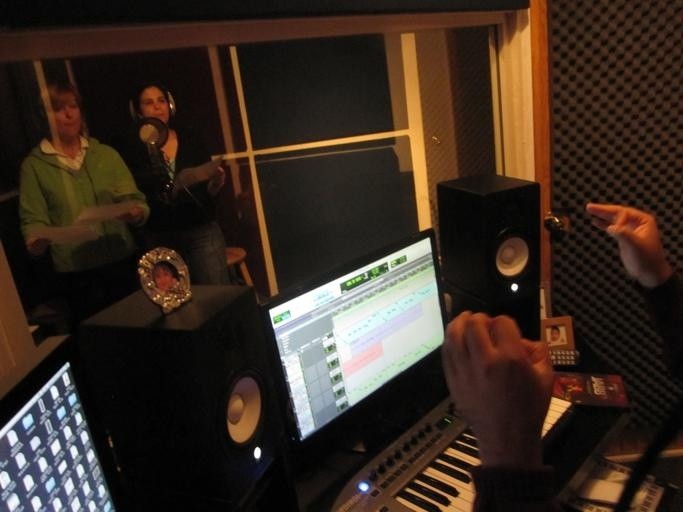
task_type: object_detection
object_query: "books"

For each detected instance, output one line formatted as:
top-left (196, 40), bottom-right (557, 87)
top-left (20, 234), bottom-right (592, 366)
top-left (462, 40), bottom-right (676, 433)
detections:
top-left (555, 370), bottom-right (632, 408)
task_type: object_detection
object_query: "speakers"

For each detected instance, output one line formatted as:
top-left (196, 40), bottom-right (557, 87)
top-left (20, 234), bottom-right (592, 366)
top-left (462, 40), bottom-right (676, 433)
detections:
top-left (435, 174), bottom-right (541, 342)
top-left (84, 283), bottom-right (279, 508)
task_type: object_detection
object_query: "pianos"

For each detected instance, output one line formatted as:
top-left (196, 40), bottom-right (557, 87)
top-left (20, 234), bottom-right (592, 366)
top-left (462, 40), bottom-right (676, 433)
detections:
top-left (329, 394), bottom-right (574, 512)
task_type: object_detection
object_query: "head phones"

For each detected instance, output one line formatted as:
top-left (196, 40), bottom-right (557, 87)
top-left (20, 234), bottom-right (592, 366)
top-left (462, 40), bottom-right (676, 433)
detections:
top-left (128, 83), bottom-right (175, 118)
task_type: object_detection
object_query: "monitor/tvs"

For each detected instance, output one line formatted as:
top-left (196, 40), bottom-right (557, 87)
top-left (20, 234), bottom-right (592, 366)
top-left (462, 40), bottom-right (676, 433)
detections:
top-left (1, 336), bottom-right (120, 511)
top-left (256, 227), bottom-right (447, 448)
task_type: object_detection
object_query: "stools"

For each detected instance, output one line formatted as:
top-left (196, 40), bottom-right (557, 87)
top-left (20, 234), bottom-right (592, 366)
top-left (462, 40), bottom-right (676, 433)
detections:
top-left (221, 245), bottom-right (260, 306)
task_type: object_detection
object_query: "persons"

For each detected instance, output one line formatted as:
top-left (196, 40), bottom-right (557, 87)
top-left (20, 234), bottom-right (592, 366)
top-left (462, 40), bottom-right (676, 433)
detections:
top-left (130, 80), bottom-right (226, 284)
top-left (20, 81), bottom-right (150, 314)
top-left (152, 262), bottom-right (180, 290)
top-left (549, 328), bottom-right (562, 345)
top-left (441, 203), bottom-right (682, 512)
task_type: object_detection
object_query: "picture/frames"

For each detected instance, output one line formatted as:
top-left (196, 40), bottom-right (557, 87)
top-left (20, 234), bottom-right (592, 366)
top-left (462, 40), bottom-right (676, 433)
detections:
top-left (137, 247), bottom-right (193, 315)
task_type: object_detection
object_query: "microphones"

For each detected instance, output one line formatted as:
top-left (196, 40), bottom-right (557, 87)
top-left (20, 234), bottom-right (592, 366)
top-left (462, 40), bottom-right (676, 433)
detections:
top-left (147, 140), bottom-right (163, 183)
